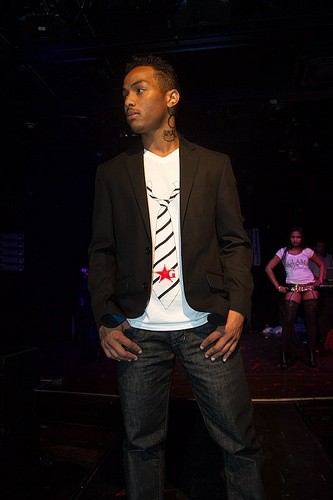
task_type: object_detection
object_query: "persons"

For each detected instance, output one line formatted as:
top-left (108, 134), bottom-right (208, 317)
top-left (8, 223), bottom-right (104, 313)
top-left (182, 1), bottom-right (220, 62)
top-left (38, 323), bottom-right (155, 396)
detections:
top-left (265, 228), bottom-right (325, 372)
top-left (308, 241), bottom-right (333, 343)
top-left (86, 53), bottom-right (263, 500)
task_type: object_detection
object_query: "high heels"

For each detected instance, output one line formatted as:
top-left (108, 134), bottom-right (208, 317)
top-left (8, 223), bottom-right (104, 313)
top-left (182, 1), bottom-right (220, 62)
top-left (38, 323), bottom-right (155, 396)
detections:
top-left (279, 351), bottom-right (288, 367)
top-left (308, 351), bottom-right (316, 366)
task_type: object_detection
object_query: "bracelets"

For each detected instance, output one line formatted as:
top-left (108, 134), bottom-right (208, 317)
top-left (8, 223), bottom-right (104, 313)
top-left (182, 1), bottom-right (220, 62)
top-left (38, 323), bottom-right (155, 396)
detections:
top-left (278, 285), bottom-right (283, 291)
